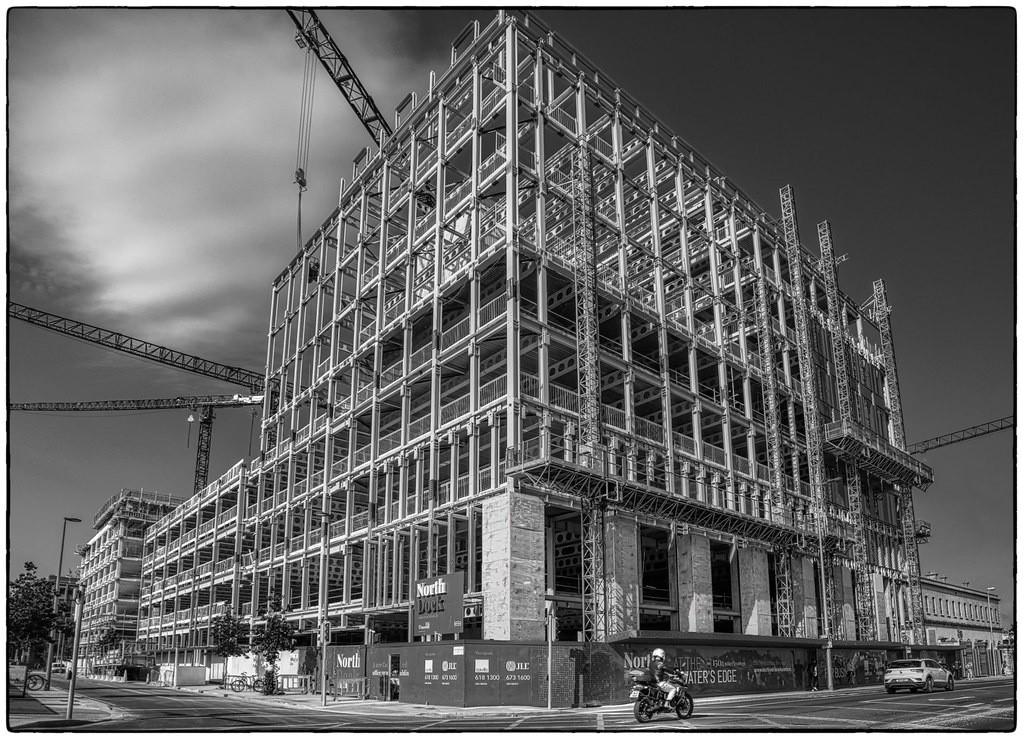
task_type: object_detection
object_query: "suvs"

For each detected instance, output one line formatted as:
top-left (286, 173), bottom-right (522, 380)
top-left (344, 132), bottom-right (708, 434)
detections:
top-left (884, 658), bottom-right (956, 695)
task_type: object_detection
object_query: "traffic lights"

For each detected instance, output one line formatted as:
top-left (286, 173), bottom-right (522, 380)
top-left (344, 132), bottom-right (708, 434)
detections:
top-left (317, 624), bottom-right (324, 644)
top-left (323, 622), bottom-right (331, 645)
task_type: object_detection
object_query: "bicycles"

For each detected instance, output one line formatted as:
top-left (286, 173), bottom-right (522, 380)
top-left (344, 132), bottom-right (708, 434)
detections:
top-left (9, 666), bottom-right (45, 691)
top-left (231, 671), bottom-right (266, 692)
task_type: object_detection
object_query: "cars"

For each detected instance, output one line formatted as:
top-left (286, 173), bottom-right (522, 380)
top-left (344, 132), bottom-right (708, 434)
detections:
top-left (51, 662), bottom-right (66, 674)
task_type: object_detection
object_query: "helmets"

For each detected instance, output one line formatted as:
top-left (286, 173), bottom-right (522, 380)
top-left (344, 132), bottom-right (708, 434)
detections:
top-left (652, 648), bottom-right (665, 660)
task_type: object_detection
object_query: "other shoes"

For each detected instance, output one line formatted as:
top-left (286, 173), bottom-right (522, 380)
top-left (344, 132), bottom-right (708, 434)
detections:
top-left (664, 701), bottom-right (673, 709)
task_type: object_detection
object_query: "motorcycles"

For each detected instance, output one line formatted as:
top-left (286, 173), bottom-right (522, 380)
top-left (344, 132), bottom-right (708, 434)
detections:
top-left (628, 661), bottom-right (694, 724)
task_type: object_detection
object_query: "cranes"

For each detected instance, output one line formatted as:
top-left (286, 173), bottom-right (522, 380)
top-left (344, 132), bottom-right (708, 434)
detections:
top-left (7, 396), bottom-right (266, 496)
top-left (9, 298), bottom-right (344, 452)
top-left (906, 413), bottom-right (1014, 454)
top-left (287, 6), bottom-right (436, 215)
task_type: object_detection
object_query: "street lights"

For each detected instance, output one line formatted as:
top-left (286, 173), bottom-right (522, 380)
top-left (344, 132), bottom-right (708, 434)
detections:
top-left (986, 587), bottom-right (997, 677)
top-left (43, 516), bottom-right (83, 689)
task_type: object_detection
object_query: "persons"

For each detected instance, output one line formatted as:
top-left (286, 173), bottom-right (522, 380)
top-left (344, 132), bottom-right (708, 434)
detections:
top-left (846, 658), bottom-right (884, 689)
top-left (1002, 660), bottom-right (1007, 676)
top-left (952, 660), bottom-right (973, 681)
top-left (794, 658), bottom-right (819, 691)
top-left (649, 648), bottom-right (684, 709)
